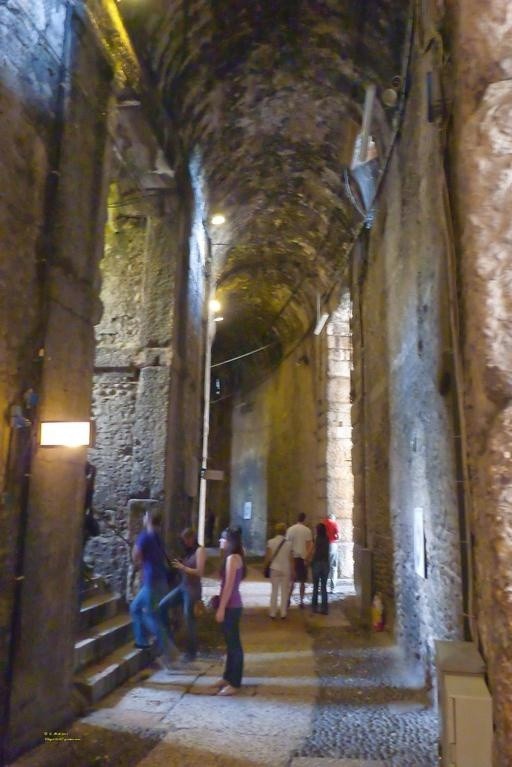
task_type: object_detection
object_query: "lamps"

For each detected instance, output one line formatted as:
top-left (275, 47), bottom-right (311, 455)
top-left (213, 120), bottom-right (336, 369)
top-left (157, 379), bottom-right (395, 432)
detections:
top-left (117, 79), bottom-right (141, 107)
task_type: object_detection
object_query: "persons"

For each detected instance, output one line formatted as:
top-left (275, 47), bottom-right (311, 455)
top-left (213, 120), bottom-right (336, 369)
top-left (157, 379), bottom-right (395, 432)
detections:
top-left (303, 523), bottom-right (330, 615)
top-left (263, 523), bottom-right (297, 619)
top-left (212, 524), bottom-right (247, 695)
top-left (129, 508), bottom-right (165, 648)
top-left (205, 508), bottom-right (215, 547)
top-left (159, 527), bottom-right (208, 663)
top-left (318, 514), bottom-right (340, 593)
top-left (286, 512), bottom-right (313, 609)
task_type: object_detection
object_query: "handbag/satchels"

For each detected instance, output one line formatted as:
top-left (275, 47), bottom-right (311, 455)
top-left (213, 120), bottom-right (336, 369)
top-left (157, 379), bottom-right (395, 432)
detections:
top-left (264, 567), bottom-right (270, 578)
top-left (207, 596), bottom-right (220, 610)
